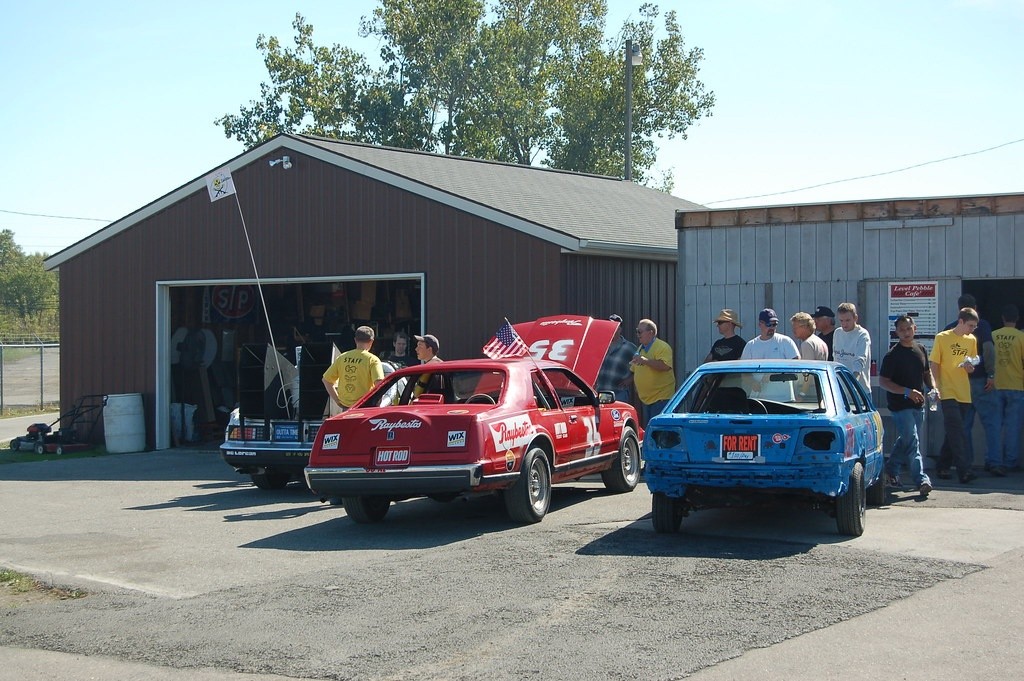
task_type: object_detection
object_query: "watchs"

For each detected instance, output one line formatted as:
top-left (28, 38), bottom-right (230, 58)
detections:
top-left (987, 374), bottom-right (994, 378)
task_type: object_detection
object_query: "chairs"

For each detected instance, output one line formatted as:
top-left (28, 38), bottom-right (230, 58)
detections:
top-left (716, 386), bottom-right (748, 414)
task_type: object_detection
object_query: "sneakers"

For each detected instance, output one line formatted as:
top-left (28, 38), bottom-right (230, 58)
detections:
top-left (884, 474), bottom-right (903, 488)
top-left (920, 482), bottom-right (931, 496)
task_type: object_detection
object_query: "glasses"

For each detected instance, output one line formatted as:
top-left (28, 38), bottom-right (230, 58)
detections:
top-left (761, 322), bottom-right (777, 327)
top-left (718, 321), bottom-right (729, 325)
top-left (636, 329), bottom-right (647, 334)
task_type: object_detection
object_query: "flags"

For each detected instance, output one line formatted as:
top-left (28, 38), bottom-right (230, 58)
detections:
top-left (481, 321), bottom-right (529, 361)
top-left (205, 168), bottom-right (235, 202)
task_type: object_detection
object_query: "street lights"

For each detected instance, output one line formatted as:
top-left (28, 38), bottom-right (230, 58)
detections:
top-left (622, 40), bottom-right (644, 180)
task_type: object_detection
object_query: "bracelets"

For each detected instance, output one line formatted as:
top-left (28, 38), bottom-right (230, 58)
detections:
top-left (904, 387), bottom-right (910, 399)
top-left (798, 391), bottom-right (807, 398)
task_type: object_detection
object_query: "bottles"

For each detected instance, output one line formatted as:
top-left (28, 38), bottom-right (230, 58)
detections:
top-left (929, 389), bottom-right (938, 411)
top-left (872, 360), bottom-right (877, 375)
top-left (748, 383), bottom-right (761, 399)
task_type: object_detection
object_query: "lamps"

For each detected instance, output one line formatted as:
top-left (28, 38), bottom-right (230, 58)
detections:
top-left (268, 156), bottom-right (292, 169)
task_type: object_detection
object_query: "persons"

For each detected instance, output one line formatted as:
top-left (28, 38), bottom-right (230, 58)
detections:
top-left (322, 326), bottom-right (384, 413)
top-left (410, 333), bottom-right (444, 405)
top-left (380, 331), bottom-right (419, 368)
top-left (928, 293), bottom-right (996, 483)
top-left (594, 313), bottom-right (639, 406)
top-left (618, 319), bottom-right (675, 430)
top-left (984, 304), bottom-right (1024, 471)
top-left (879, 317), bottom-right (941, 497)
top-left (704, 303), bottom-right (872, 403)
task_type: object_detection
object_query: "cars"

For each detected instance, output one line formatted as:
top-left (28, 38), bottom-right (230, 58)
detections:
top-left (302, 356), bottom-right (646, 526)
top-left (641, 357), bottom-right (888, 537)
top-left (219, 360), bottom-right (416, 492)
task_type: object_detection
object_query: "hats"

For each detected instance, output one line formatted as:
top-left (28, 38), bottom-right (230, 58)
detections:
top-left (810, 306), bottom-right (835, 317)
top-left (413, 334), bottom-right (439, 352)
top-left (713, 309), bottom-right (742, 329)
top-left (606, 313), bottom-right (623, 327)
top-left (758, 309), bottom-right (779, 321)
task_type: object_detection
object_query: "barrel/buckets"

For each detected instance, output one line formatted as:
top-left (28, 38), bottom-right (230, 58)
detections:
top-left (103, 392), bottom-right (146, 454)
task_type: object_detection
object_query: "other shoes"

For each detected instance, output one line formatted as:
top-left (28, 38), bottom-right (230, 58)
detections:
top-left (960, 470), bottom-right (968, 481)
top-left (990, 467), bottom-right (1006, 477)
top-left (937, 467), bottom-right (951, 478)
